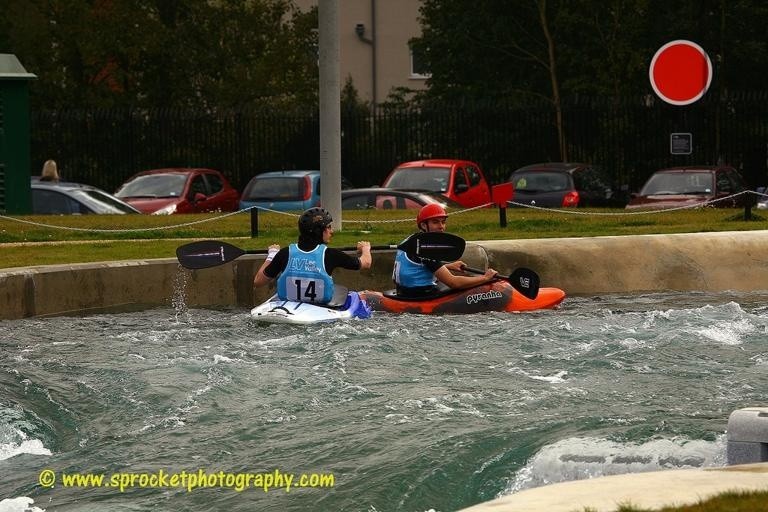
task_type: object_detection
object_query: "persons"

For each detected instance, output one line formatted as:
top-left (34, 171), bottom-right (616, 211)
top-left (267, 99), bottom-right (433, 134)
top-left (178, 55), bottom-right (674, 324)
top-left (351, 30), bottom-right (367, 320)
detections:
top-left (391, 203), bottom-right (499, 299)
top-left (254, 206), bottom-right (372, 309)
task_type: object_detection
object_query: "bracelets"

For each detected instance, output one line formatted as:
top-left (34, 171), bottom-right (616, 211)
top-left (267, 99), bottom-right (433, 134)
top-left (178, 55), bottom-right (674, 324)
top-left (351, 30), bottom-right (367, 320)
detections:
top-left (265, 249), bottom-right (280, 261)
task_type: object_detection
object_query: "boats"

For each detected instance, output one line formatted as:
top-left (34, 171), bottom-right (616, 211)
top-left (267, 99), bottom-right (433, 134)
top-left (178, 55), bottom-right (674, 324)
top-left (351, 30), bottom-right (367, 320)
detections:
top-left (251, 283), bottom-right (372, 326)
top-left (358, 278), bottom-right (568, 314)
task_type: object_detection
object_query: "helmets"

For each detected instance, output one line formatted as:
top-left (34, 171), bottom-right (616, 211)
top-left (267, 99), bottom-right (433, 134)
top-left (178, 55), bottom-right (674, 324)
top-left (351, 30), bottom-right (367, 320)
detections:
top-left (416, 202), bottom-right (450, 225)
top-left (296, 206), bottom-right (334, 240)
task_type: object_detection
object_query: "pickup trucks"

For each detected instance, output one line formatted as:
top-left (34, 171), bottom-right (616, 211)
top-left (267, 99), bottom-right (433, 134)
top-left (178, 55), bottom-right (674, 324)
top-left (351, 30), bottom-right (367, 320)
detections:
top-left (375, 159), bottom-right (513, 213)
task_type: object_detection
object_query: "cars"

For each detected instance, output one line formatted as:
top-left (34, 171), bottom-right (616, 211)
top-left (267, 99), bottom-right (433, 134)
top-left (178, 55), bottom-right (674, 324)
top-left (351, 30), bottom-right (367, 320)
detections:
top-left (30, 181), bottom-right (141, 216)
top-left (504, 161), bottom-right (595, 210)
top-left (238, 170), bottom-right (380, 213)
top-left (623, 167), bottom-right (748, 211)
top-left (111, 167), bottom-right (242, 215)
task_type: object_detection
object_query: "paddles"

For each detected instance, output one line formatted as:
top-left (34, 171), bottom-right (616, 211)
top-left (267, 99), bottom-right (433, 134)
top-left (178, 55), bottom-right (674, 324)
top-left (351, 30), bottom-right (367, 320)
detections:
top-left (176, 231), bottom-right (465, 270)
top-left (423, 256), bottom-right (540, 300)
